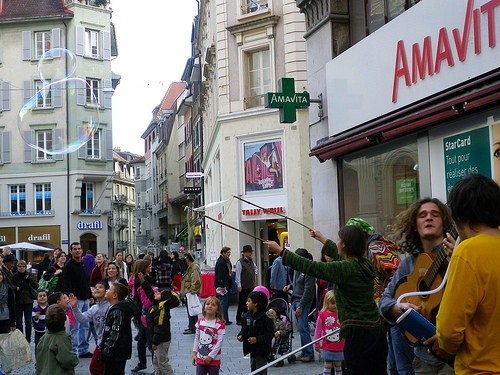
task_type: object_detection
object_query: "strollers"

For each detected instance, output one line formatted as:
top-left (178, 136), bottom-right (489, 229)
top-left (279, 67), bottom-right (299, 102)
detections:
top-left (265, 297), bottom-right (301, 368)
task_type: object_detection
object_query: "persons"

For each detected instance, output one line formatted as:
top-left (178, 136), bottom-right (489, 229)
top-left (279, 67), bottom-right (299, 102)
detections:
top-left (34, 304), bottom-right (81, 375)
top-left (290, 246), bottom-right (315, 364)
top-left (98, 277), bottom-right (133, 374)
top-left (423, 172), bottom-right (500, 375)
top-left (213, 246), bottom-right (234, 326)
top-left (345, 216), bottom-right (415, 375)
top-left (235, 290), bottom-right (275, 375)
top-left (191, 296), bottom-right (227, 375)
top-left (251, 150), bottom-right (281, 181)
top-left (312, 289), bottom-right (346, 375)
top-left (261, 223), bottom-right (390, 375)
top-left (267, 306), bottom-right (288, 360)
top-left (180, 252), bottom-right (202, 334)
top-left (235, 244), bottom-right (259, 326)
top-left (0, 241), bottom-right (189, 372)
top-left (137, 270), bottom-right (176, 374)
top-left (316, 248), bottom-right (337, 313)
top-left (379, 196), bottom-right (460, 375)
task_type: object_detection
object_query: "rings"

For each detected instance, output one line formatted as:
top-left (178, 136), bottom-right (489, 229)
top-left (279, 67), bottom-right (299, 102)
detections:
top-left (444, 245), bottom-right (447, 250)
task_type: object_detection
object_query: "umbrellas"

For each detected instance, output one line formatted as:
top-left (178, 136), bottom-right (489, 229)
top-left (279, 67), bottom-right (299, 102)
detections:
top-left (0, 241), bottom-right (55, 260)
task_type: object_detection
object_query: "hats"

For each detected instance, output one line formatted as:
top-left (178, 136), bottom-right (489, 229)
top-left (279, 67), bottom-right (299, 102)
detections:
top-left (241, 245), bottom-right (255, 252)
top-left (3, 254), bottom-right (18, 264)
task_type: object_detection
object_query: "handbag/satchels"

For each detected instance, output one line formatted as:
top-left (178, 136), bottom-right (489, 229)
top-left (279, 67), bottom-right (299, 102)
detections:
top-left (0, 329), bottom-right (33, 372)
top-left (28, 283), bottom-right (38, 300)
top-left (186, 292), bottom-right (203, 317)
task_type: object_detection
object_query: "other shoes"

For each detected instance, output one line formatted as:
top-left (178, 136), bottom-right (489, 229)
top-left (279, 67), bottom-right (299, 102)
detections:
top-left (301, 356), bottom-right (314, 362)
top-left (131, 363), bottom-right (147, 371)
top-left (79, 352), bottom-right (93, 358)
top-left (184, 329), bottom-right (189, 331)
top-left (296, 354), bottom-right (304, 361)
top-left (225, 321), bottom-right (232, 325)
top-left (183, 330), bottom-right (196, 334)
